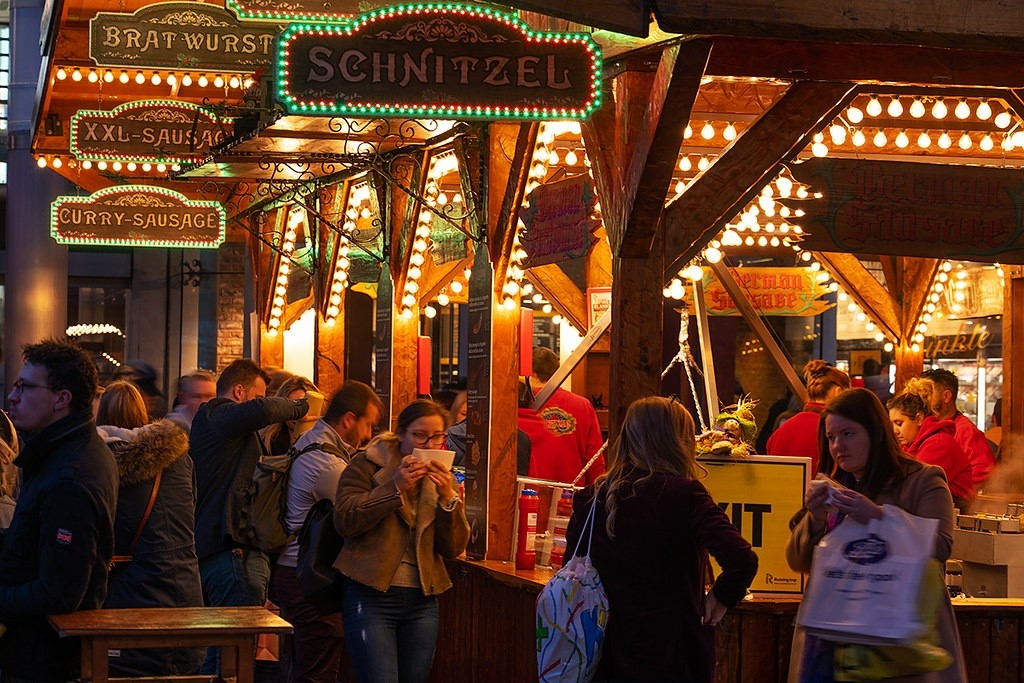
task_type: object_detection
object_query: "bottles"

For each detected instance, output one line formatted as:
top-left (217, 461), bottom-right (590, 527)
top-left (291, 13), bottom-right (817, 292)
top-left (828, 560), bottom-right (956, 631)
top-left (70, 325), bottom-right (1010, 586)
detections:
top-left (294, 391), bottom-right (324, 439)
top-left (550, 493), bottom-right (573, 570)
top-left (945, 560), bottom-right (963, 598)
top-left (515, 489), bottom-right (539, 570)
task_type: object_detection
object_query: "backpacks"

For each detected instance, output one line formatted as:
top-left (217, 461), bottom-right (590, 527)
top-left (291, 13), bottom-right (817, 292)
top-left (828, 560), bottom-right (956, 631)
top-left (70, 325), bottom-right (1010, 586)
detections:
top-left (225, 434), bottom-right (350, 553)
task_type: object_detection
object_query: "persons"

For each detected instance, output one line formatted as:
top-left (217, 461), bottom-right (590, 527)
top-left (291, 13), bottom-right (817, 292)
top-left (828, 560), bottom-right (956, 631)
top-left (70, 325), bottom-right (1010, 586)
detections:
top-left (562, 395), bottom-right (759, 683)
top-left (784, 389), bottom-right (969, 683)
top-left (331, 400), bottom-right (472, 683)
top-left (268, 382), bottom-right (385, 683)
top-left (431, 378), bottom-right (468, 467)
top-left (763, 360), bottom-right (852, 479)
top-left (516, 346), bottom-right (606, 534)
top-left (0, 357), bottom-right (326, 683)
top-left (0, 333), bottom-right (121, 683)
top-left (888, 368), bottom-right (1002, 514)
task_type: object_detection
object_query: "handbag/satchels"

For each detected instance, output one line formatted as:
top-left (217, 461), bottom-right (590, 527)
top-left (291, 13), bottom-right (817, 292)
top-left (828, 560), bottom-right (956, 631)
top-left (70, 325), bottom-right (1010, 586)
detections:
top-left (536, 476), bottom-right (609, 683)
top-left (834, 557), bottom-right (954, 683)
top-left (297, 498), bottom-right (347, 611)
top-left (796, 504), bottom-right (941, 646)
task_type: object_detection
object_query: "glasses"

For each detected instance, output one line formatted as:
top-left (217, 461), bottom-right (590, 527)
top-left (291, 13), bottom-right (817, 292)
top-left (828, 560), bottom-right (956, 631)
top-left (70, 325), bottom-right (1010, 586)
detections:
top-left (13, 380), bottom-right (57, 394)
top-left (406, 428), bottom-right (448, 446)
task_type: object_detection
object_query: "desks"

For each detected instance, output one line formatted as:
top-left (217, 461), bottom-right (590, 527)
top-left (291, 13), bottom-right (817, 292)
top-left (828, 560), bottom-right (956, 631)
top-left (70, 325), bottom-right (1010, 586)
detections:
top-left (46, 606), bottom-right (295, 683)
